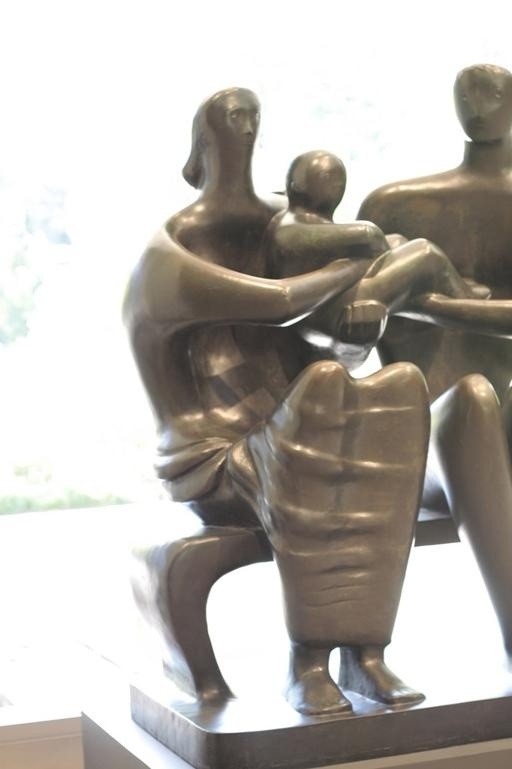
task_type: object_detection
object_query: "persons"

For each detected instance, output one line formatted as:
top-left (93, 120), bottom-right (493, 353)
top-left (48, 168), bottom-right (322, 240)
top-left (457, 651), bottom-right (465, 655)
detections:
top-left (260, 143), bottom-right (493, 345)
top-left (120, 85), bottom-right (427, 718)
top-left (356, 65), bottom-right (512, 679)
